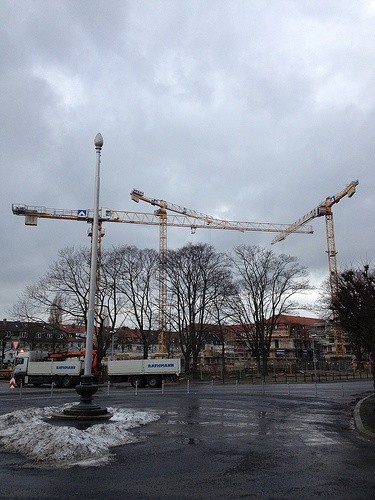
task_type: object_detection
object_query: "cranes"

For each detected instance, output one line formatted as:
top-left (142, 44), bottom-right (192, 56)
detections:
top-left (9, 202), bottom-right (316, 367)
top-left (129, 188), bottom-right (245, 362)
top-left (269, 177), bottom-right (359, 358)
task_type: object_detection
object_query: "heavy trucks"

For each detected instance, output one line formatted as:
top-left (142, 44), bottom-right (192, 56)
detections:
top-left (11, 348), bottom-right (182, 389)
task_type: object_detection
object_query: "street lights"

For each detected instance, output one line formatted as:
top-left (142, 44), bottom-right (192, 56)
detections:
top-left (75, 132), bottom-right (103, 403)
